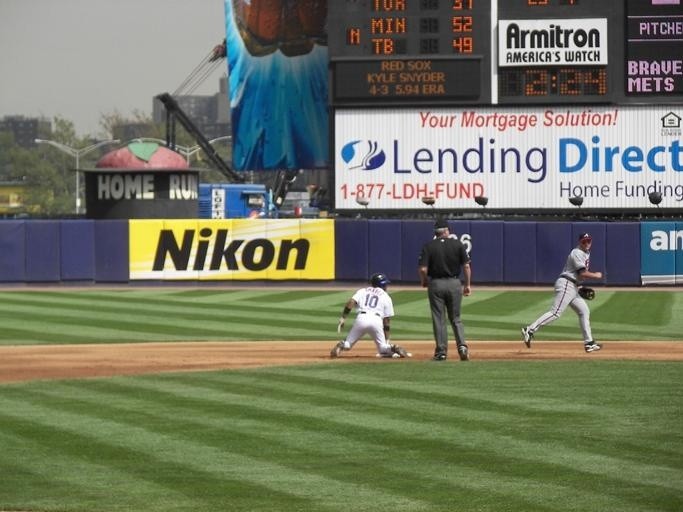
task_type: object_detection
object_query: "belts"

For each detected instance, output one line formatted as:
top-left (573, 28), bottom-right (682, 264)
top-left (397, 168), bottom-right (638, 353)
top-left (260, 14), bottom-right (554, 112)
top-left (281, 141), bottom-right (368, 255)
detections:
top-left (561, 274), bottom-right (576, 285)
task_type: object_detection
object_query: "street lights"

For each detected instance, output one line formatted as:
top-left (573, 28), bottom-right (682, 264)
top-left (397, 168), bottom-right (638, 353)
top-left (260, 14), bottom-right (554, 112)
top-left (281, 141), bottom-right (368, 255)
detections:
top-left (134, 136), bottom-right (233, 168)
top-left (35, 139), bottom-right (120, 214)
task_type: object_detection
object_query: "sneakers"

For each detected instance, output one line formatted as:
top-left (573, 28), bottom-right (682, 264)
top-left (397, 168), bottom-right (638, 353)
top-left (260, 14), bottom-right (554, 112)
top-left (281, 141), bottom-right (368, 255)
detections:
top-left (584, 340), bottom-right (601, 353)
top-left (458, 344), bottom-right (470, 360)
top-left (391, 345), bottom-right (407, 358)
top-left (433, 352), bottom-right (447, 361)
top-left (520, 326), bottom-right (534, 349)
top-left (331, 342), bottom-right (345, 359)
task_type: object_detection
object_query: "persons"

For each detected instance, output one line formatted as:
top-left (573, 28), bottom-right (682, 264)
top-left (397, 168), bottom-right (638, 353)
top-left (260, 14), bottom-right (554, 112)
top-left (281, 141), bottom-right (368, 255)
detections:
top-left (520, 232), bottom-right (603, 354)
top-left (416, 218), bottom-right (471, 361)
top-left (328, 272), bottom-right (407, 360)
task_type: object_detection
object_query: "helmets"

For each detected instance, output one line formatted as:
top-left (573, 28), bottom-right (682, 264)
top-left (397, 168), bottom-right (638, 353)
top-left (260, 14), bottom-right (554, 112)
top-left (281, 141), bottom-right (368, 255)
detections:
top-left (370, 273), bottom-right (390, 288)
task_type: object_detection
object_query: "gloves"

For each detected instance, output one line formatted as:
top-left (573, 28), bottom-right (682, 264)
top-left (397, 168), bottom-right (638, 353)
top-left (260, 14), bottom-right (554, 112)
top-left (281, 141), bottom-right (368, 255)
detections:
top-left (337, 317), bottom-right (345, 334)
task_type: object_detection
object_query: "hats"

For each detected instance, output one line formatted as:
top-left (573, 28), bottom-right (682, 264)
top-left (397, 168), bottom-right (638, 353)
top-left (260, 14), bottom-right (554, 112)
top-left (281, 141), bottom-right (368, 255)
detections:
top-left (434, 219), bottom-right (450, 230)
top-left (578, 231), bottom-right (594, 243)
